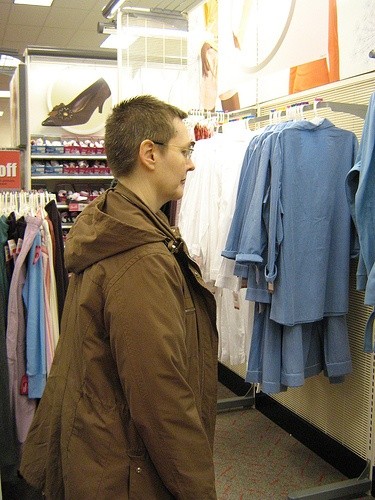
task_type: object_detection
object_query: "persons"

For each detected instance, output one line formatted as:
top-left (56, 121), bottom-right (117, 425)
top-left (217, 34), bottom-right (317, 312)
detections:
top-left (198, 0), bottom-right (241, 119)
top-left (17, 95), bottom-right (218, 500)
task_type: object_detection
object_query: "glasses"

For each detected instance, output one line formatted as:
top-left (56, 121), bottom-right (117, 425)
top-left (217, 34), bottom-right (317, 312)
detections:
top-left (151, 141), bottom-right (194, 161)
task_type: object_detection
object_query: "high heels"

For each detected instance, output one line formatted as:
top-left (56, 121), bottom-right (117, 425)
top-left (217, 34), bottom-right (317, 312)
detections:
top-left (41, 76), bottom-right (112, 127)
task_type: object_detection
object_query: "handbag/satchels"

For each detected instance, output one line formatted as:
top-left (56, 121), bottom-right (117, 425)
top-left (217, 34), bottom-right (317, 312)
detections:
top-left (287, 57), bottom-right (329, 95)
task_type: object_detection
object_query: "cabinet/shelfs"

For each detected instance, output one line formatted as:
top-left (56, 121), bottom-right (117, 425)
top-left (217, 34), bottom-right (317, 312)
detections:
top-left (27, 135), bottom-right (114, 249)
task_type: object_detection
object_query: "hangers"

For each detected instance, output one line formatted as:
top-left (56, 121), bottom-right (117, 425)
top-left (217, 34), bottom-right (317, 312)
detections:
top-left (0, 190), bottom-right (56, 220)
top-left (186, 96), bottom-right (323, 141)
